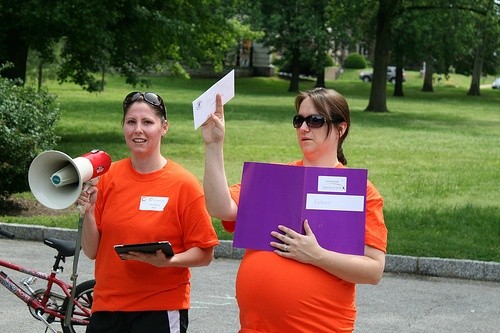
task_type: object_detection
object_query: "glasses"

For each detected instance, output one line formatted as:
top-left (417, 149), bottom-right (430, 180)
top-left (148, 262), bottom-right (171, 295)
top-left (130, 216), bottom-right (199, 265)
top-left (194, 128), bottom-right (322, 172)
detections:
top-left (293, 114), bottom-right (339, 130)
top-left (122, 92), bottom-right (166, 116)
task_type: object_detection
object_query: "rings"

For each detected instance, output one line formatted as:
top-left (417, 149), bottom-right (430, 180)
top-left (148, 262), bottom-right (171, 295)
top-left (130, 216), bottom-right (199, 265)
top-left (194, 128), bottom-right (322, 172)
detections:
top-left (285, 245), bottom-right (288, 251)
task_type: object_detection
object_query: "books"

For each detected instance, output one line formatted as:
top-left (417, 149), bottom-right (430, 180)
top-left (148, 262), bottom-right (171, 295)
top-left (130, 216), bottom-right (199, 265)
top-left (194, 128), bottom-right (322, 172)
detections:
top-left (232, 161), bottom-right (368, 256)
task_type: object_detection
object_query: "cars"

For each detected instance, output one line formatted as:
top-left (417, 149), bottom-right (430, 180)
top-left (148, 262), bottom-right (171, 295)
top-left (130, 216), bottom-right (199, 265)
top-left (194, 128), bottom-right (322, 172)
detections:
top-left (492, 78), bottom-right (500, 89)
top-left (359, 65), bottom-right (406, 83)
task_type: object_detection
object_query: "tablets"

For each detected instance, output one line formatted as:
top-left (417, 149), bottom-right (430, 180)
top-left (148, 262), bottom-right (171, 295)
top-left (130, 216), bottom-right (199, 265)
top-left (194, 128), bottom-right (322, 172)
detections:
top-left (113, 241), bottom-right (174, 260)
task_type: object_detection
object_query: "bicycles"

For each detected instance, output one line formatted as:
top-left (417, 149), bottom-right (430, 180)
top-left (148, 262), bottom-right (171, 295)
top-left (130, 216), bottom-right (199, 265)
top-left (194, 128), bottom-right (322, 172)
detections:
top-left (0, 230), bottom-right (96, 333)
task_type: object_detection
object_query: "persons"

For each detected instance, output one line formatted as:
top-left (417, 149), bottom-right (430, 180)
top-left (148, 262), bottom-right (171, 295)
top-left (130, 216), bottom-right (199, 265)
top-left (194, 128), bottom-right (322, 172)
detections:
top-left (200, 88), bottom-right (388, 333)
top-left (74, 91), bottom-right (219, 333)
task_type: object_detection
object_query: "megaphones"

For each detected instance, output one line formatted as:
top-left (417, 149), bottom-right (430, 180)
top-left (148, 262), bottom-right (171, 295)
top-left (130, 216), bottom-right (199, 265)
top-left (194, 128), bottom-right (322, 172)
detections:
top-left (28, 150), bottom-right (111, 215)
